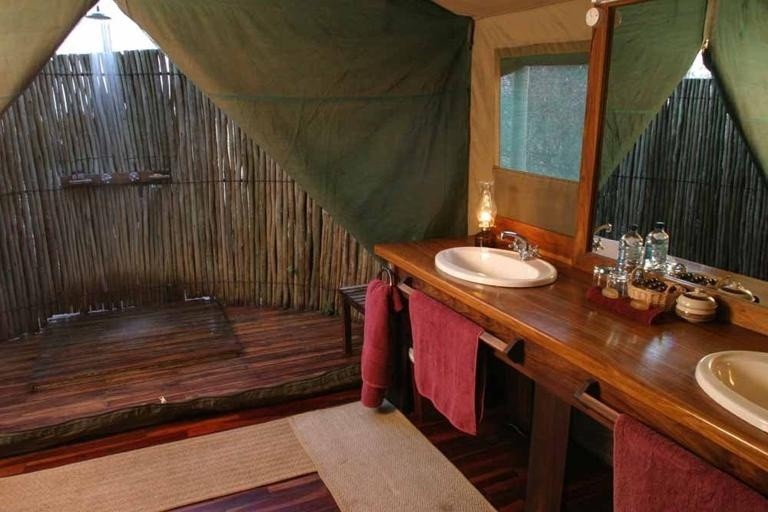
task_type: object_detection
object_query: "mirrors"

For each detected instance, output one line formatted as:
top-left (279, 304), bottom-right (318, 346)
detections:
top-left (573, 0), bottom-right (767, 338)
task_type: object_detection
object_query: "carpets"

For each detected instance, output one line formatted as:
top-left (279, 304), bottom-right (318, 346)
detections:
top-left (0, 416), bottom-right (319, 512)
top-left (287, 397), bottom-right (499, 512)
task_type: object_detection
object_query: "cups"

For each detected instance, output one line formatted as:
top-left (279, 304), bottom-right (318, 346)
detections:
top-left (593, 267), bottom-right (629, 298)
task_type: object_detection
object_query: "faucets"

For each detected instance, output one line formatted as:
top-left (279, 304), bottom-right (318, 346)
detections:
top-left (593, 224), bottom-right (614, 237)
top-left (500, 230), bottom-right (529, 250)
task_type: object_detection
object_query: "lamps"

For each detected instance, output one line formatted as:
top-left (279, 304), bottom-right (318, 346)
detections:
top-left (475, 181), bottom-right (497, 247)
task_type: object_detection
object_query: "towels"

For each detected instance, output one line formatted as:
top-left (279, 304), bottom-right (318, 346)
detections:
top-left (612, 414), bottom-right (768, 512)
top-left (585, 286), bottom-right (664, 326)
top-left (409, 288), bottom-right (486, 436)
top-left (359, 280), bottom-right (403, 408)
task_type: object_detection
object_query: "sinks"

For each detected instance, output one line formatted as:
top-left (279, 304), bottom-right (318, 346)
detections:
top-left (697, 349), bottom-right (768, 434)
top-left (435, 246), bottom-right (559, 287)
